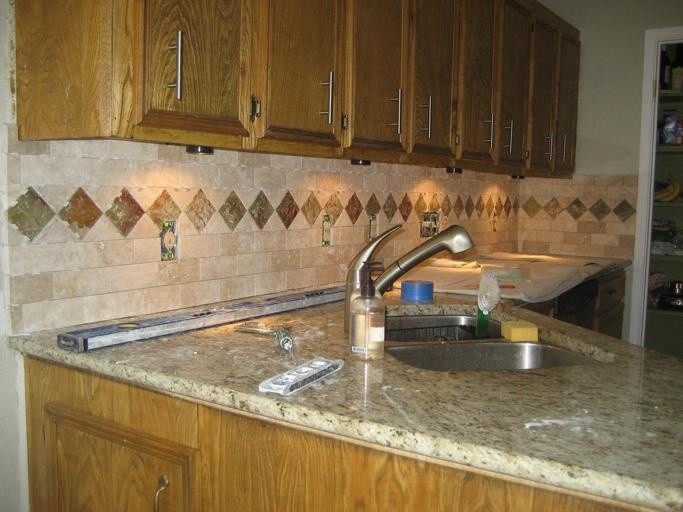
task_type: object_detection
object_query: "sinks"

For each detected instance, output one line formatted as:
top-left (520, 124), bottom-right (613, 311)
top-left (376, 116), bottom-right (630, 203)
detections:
top-left (385, 342), bottom-right (604, 373)
top-left (384, 316), bottom-right (509, 343)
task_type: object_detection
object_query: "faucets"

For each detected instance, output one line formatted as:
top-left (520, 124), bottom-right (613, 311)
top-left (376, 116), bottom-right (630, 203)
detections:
top-left (344, 224), bottom-right (473, 335)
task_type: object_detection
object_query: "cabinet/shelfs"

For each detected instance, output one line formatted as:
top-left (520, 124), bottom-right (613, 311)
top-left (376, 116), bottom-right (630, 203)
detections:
top-left (249, 0), bottom-right (343, 159)
top-left (455, 0), bottom-right (525, 177)
top-left (646, 91), bottom-right (683, 318)
top-left (526, 0), bottom-right (581, 180)
top-left (43, 402), bottom-right (202, 511)
top-left (14, 1), bottom-right (247, 152)
top-left (345, 0), bottom-right (454, 167)
top-left (518, 270), bottom-right (626, 342)
